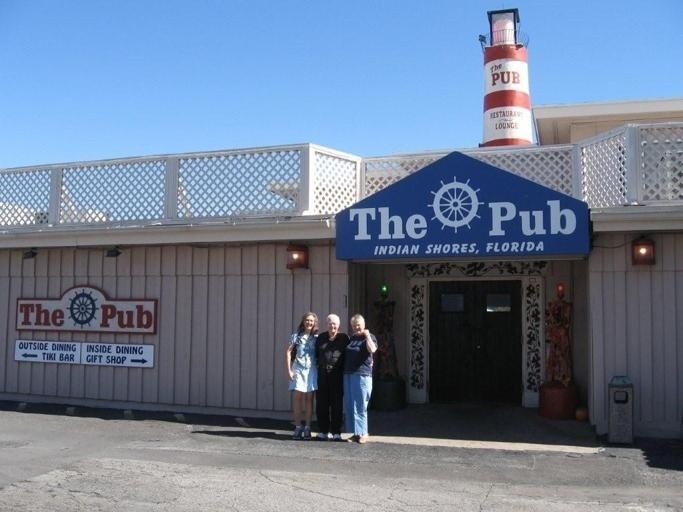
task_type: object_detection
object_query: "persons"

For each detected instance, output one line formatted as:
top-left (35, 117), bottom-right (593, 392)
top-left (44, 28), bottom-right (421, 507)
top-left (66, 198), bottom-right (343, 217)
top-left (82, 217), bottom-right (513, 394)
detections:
top-left (316, 313), bottom-right (351, 442)
top-left (343, 312), bottom-right (380, 445)
top-left (285, 311), bottom-right (320, 441)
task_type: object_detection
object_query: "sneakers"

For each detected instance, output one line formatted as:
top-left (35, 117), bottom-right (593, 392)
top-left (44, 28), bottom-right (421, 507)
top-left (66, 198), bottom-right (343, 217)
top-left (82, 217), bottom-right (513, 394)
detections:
top-left (293, 425), bottom-right (370, 444)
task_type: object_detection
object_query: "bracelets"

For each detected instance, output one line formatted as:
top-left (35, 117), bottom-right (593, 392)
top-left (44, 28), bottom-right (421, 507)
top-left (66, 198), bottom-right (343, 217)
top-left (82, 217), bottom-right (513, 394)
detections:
top-left (364, 332), bottom-right (370, 338)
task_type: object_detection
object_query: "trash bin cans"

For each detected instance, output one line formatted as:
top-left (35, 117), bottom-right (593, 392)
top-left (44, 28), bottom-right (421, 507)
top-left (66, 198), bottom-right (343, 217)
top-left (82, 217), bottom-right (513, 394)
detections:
top-left (607, 375), bottom-right (634, 444)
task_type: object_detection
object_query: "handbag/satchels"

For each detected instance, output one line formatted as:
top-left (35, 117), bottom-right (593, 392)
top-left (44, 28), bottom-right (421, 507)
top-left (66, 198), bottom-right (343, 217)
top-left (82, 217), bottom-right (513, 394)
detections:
top-left (291, 345), bottom-right (297, 361)
